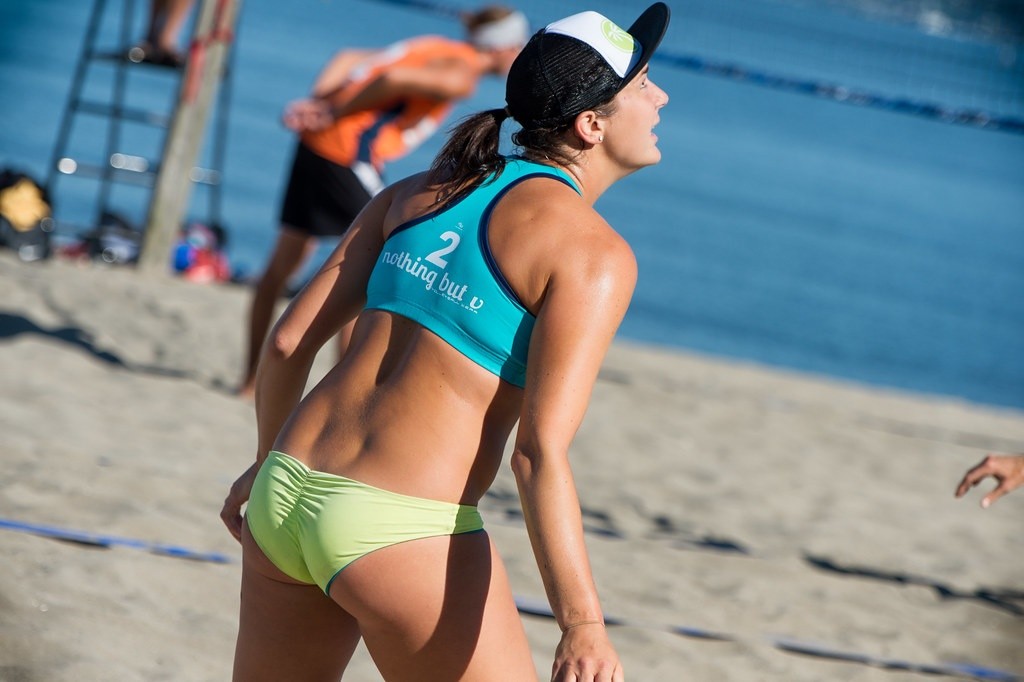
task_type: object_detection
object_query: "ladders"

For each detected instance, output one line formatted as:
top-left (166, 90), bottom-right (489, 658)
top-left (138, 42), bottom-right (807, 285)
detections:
top-left (45, 0), bottom-right (241, 279)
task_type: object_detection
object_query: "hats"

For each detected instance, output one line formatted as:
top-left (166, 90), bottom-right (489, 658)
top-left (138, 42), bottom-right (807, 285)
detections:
top-left (506, 2), bottom-right (670, 124)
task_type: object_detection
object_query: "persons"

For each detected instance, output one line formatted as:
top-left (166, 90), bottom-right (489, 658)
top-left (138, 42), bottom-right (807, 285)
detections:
top-left (129, 0), bottom-right (193, 66)
top-left (219, 3), bottom-right (672, 682)
top-left (955, 454), bottom-right (1024, 507)
top-left (233, 9), bottom-right (531, 399)
